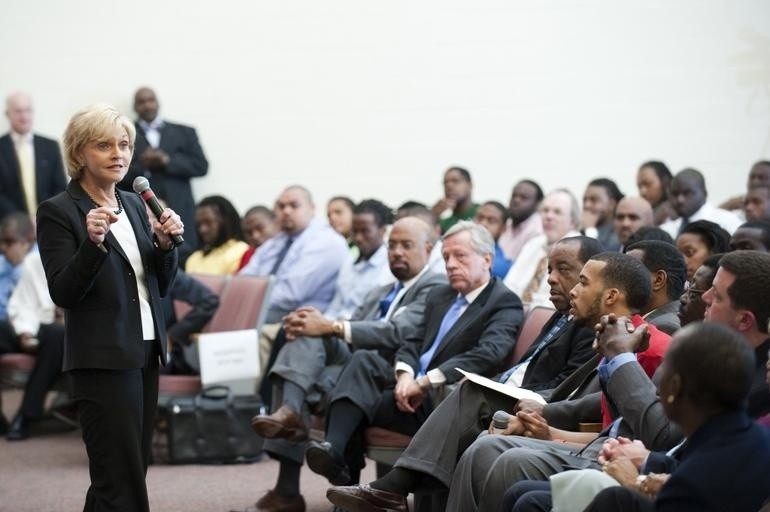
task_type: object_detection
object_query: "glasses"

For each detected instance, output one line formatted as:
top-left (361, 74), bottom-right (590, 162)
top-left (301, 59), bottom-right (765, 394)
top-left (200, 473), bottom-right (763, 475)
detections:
top-left (687, 284), bottom-right (709, 298)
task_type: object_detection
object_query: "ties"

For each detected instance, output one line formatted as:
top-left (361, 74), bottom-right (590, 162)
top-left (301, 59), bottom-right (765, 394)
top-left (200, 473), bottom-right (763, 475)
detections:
top-left (415, 296), bottom-right (469, 376)
top-left (521, 246), bottom-right (554, 304)
top-left (378, 283), bottom-right (405, 316)
top-left (16, 137), bottom-right (38, 216)
top-left (499, 314), bottom-right (569, 384)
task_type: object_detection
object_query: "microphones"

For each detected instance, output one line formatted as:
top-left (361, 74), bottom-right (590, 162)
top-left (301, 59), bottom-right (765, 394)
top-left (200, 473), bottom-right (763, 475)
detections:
top-left (492, 410), bottom-right (509, 434)
top-left (132, 175), bottom-right (185, 247)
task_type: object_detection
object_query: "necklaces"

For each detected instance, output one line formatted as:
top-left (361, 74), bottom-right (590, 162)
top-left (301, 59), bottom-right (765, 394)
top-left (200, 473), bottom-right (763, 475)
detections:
top-left (88, 190), bottom-right (124, 215)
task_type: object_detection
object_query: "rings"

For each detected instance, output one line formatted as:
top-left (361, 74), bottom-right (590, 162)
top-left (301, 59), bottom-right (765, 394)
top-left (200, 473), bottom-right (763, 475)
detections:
top-left (95, 219), bottom-right (102, 225)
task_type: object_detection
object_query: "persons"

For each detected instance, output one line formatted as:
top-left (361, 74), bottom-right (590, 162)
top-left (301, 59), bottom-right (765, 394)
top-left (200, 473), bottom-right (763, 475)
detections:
top-left (34, 104), bottom-right (185, 512)
top-left (176, 159), bottom-right (769, 512)
top-left (5, 243), bottom-right (66, 442)
top-left (1, 94), bottom-right (69, 241)
top-left (1, 210), bottom-right (43, 440)
top-left (111, 84), bottom-right (207, 276)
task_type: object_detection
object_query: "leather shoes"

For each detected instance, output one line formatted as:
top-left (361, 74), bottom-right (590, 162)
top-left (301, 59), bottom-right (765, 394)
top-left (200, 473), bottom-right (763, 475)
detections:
top-left (7, 413), bottom-right (28, 440)
top-left (326, 484), bottom-right (409, 511)
top-left (250, 404), bottom-right (309, 444)
top-left (305, 439), bottom-right (353, 485)
top-left (234, 488), bottom-right (306, 512)
